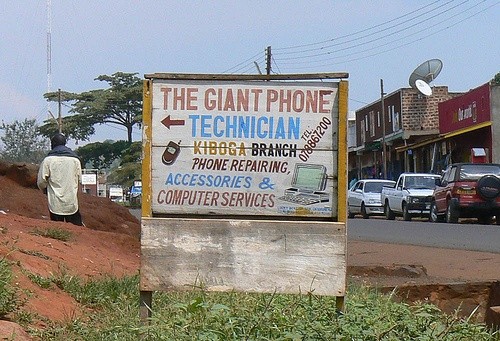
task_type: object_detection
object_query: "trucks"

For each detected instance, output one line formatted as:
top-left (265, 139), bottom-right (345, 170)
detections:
top-left (108, 185), bottom-right (123, 203)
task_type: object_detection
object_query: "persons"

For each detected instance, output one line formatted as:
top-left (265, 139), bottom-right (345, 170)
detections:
top-left (36, 133), bottom-right (83, 225)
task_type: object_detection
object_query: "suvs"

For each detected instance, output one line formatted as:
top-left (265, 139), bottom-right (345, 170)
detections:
top-left (429, 162), bottom-right (500, 226)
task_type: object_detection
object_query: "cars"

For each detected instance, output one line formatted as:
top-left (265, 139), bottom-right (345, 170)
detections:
top-left (130, 184), bottom-right (142, 194)
top-left (348, 179), bottom-right (396, 219)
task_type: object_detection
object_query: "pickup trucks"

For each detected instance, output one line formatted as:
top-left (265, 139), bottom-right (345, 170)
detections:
top-left (381, 172), bottom-right (443, 220)
